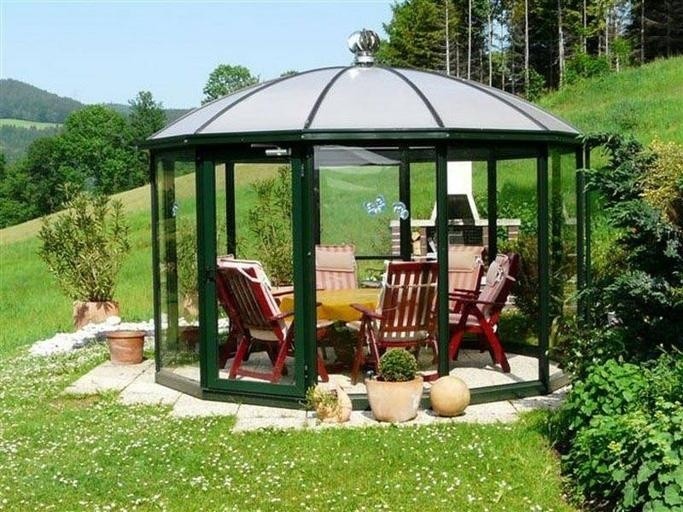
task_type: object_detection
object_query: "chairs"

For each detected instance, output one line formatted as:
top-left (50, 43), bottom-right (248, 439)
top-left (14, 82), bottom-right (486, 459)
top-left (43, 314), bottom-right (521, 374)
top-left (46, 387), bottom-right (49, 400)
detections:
top-left (216, 245), bottom-right (518, 386)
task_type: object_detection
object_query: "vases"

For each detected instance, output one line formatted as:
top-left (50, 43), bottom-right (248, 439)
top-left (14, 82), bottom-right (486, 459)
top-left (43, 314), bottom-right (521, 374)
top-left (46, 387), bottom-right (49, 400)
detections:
top-left (105, 330), bottom-right (146, 364)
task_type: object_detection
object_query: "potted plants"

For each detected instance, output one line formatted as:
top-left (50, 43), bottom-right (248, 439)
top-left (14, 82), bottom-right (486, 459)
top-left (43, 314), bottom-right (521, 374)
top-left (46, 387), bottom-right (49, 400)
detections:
top-left (161, 216), bottom-right (198, 316)
top-left (35, 178), bottom-right (131, 327)
top-left (299, 346), bottom-right (426, 427)
